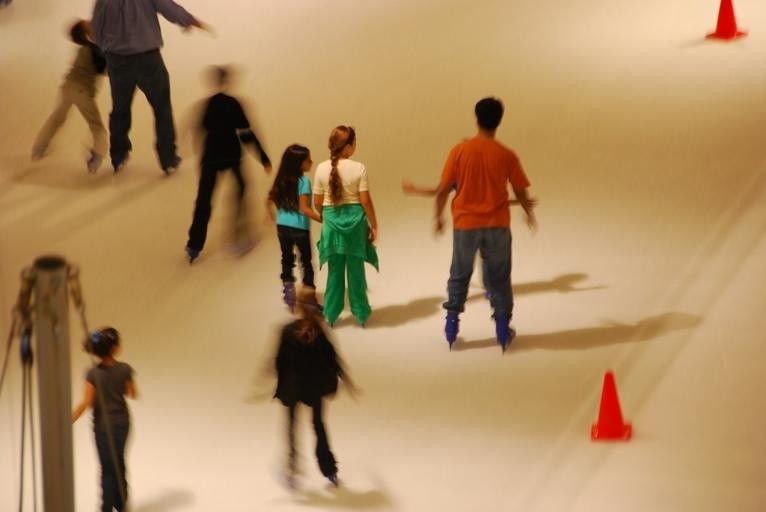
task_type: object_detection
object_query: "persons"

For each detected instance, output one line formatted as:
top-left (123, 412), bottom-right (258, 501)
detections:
top-left (274, 287), bottom-right (359, 480)
top-left (184, 66), bottom-right (272, 259)
top-left (399, 97), bottom-right (540, 354)
top-left (28, 0), bottom-right (215, 173)
top-left (72, 325), bottom-right (141, 512)
top-left (264, 125), bottom-right (379, 327)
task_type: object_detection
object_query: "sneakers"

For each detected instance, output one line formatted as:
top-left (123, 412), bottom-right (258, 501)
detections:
top-left (320, 453), bottom-right (339, 478)
top-left (184, 244), bottom-right (199, 259)
top-left (161, 155), bottom-right (182, 170)
top-left (111, 150), bottom-right (130, 168)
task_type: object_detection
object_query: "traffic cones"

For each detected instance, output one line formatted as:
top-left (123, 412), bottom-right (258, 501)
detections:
top-left (705, 0), bottom-right (747, 42)
top-left (589, 371), bottom-right (632, 440)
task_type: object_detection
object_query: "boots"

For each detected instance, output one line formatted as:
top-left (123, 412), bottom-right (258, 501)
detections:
top-left (283, 280), bottom-right (297, 307)
top-left (495, 315), bottom-right (515, 346)
top-left (444, 311), bottom-right (459, 344)
